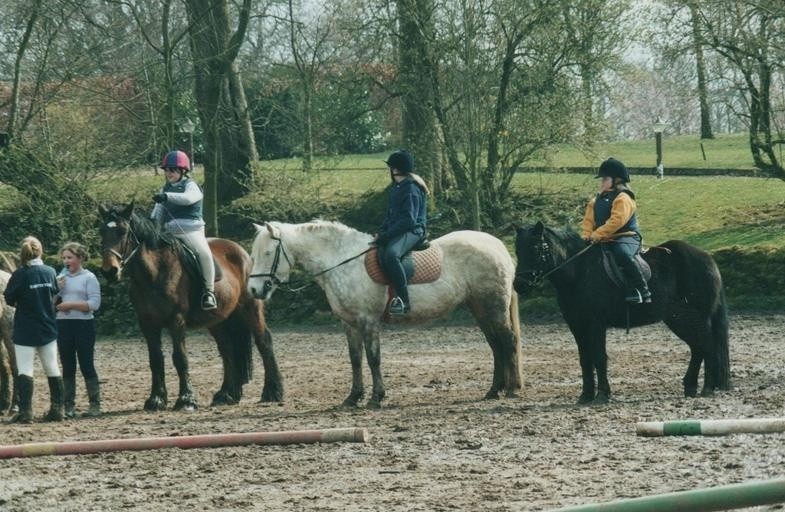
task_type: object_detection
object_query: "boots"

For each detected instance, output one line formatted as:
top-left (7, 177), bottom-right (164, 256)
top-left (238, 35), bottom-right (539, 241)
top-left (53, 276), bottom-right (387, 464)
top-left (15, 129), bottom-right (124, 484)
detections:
top-left (390, 286), bottom-right (411, 313)
top-left (63, 375), bottom-right (76, 418)
top-left (43, 376), bottom-right (64, 421)
top-left (623, 257), bottom-right (652, 304)
top-left (10, 375), bottom-right (34, 423)
top-left (85, 376), bottom-right (100, 416)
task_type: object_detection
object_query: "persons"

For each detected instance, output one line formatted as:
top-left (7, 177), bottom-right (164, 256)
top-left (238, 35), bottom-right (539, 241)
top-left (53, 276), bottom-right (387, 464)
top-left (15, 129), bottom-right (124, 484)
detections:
top-left (580, 157), bottom-right (652, 301)
top-left (3, 235), bottom-right (64, 422)
top-left (54, 242), bottom-right (102, 416)
top-left (149, 151), bottom-right (215, 308)
top-left (376, 150), bottom-right (429, 313)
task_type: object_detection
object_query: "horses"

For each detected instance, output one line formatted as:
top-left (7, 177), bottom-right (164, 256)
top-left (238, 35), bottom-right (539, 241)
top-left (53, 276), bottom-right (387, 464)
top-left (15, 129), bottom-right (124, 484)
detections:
top-left (97, 201), bottom-right (283, 410)
top-left (246, 218), bottom-right (523, 409)
top-left (513, 221), bottom-right (730, 404)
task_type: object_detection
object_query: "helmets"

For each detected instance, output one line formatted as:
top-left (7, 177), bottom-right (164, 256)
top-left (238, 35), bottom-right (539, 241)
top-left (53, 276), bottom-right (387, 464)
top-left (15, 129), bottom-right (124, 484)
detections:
top-left (382, 149), bottom-right (414, 172)
top-left (593, 156), bottom-right (630, 183)
top-left (160, 150), bottom-right (190, 171)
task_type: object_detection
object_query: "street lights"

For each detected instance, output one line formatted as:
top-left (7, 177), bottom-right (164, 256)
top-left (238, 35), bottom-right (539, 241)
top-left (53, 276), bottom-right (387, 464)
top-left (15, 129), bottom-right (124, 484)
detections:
top-left (182, 118), bottom-right (196, 170)
top-left (653, 116), bottom-right (666, 179)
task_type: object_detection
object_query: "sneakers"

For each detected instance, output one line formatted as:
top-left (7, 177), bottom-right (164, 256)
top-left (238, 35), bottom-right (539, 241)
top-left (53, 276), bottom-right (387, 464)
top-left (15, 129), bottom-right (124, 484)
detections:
top-left (203, 291), bottom-right (215, 306)
